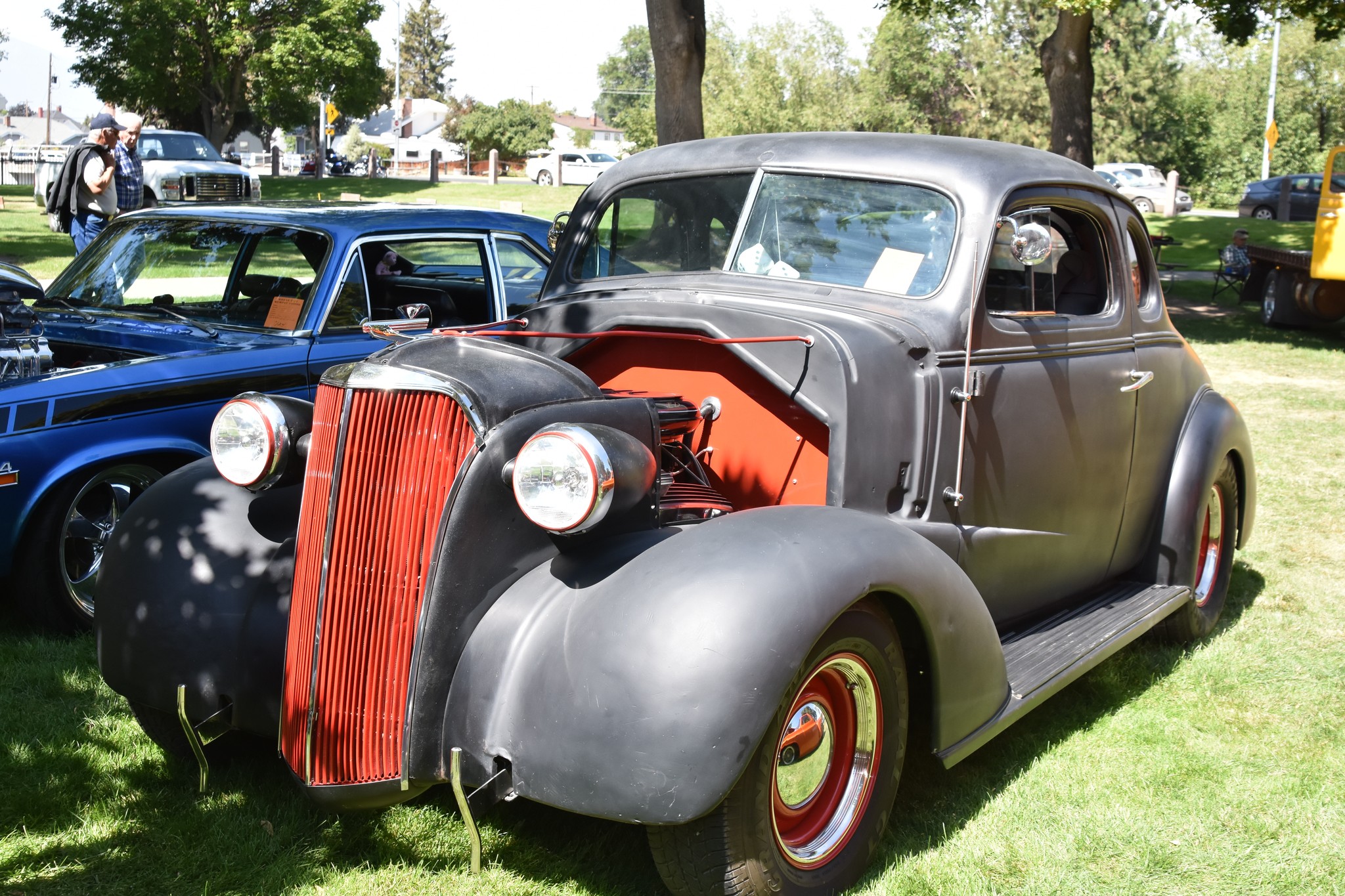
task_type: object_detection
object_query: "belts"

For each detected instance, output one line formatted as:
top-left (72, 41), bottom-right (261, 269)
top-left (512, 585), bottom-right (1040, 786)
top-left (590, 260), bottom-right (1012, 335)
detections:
top-left (77, 208), bottom-right (114, 222)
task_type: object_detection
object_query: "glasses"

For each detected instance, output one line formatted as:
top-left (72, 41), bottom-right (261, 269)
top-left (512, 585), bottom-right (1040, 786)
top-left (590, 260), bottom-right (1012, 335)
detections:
top-left (101, 128), bottom-right (119, 137)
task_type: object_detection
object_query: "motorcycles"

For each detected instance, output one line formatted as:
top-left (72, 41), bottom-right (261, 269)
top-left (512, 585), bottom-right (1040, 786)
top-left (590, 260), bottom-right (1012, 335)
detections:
top-left (296, 154), bottom-right (388, 179)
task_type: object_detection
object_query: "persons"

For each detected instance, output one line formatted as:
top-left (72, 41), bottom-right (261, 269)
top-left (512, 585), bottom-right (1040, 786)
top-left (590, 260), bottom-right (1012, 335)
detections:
top-left (65, 112), bottom-right (147, 309)
top-left (1223, 228), bottom-right (1253, 277)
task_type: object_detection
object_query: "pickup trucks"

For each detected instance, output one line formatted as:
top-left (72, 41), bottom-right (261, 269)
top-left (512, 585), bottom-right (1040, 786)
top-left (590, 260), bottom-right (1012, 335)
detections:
top-left (32, 128), bottom-right (262, 233)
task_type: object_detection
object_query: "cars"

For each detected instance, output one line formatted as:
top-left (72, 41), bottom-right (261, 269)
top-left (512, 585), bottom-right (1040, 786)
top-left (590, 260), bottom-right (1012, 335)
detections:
top-left (1091, 163), bottom-right (1194, 215)
top-left (0, 205), bottom-right (650, 633)
top-left (525, 149), bottom-right (620, 187)
top-left (1238, 173), bottom-right (1345, 221)
top-left (96, 131), bottom-right (1257, 896)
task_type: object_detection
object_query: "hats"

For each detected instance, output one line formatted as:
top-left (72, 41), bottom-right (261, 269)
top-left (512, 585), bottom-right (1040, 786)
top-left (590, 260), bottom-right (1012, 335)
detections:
top-left (88, 112), bottom-right (128, 131)
top-left (1234, 229), bottom-right (1249, 239)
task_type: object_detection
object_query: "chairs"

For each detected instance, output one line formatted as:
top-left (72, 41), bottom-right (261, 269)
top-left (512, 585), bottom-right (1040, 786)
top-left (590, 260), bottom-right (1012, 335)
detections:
top-left (1212, 249), bottom-right (1247, 300)
top-left (1150, 235), bottom-right (1189, 295)
top-left (228, 274), bottom-right (302, 322)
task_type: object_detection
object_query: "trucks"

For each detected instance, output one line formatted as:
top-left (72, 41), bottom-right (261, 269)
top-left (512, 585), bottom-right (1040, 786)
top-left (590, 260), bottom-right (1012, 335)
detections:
top-left (1244, 145), bottom-right (1345, 331)
